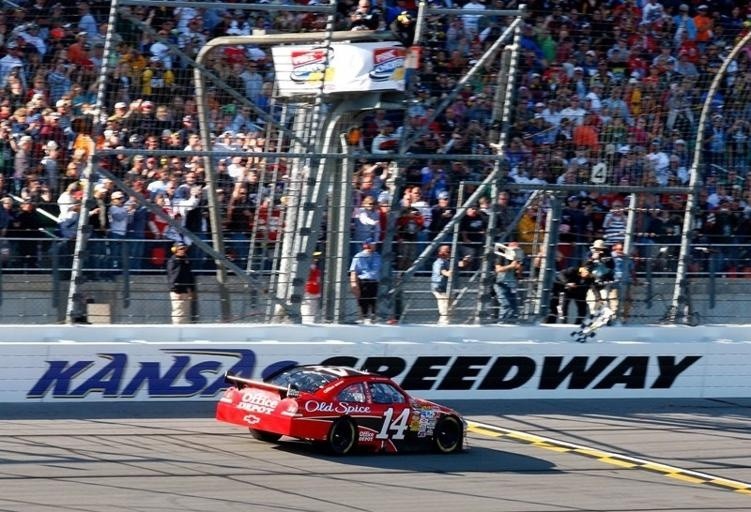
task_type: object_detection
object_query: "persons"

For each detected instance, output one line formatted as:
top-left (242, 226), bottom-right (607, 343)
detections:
top-left (0, 1), bottom-right (751, 326)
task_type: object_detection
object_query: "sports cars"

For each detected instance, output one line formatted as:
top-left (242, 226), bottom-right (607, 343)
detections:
top-left (216, 364), bottom-right (473, 458)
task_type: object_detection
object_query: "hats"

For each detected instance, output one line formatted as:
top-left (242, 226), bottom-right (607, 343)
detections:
top-left (362, 195), bottom-right (377, 206)
top-left (435, 190), bottom-right (450, 201)
top-left (567, 194), bottom-right (578, 203)
top-left (172, 241), bottom-right (188, 250)
top-left (1, 35), bottom-right (262, 202)
top-left (590, 239), bottom-right (607, 250)
top-left (363, 238), bottom-right (376, 245)
top-left (514, 4), bottom-right (722, 164)
